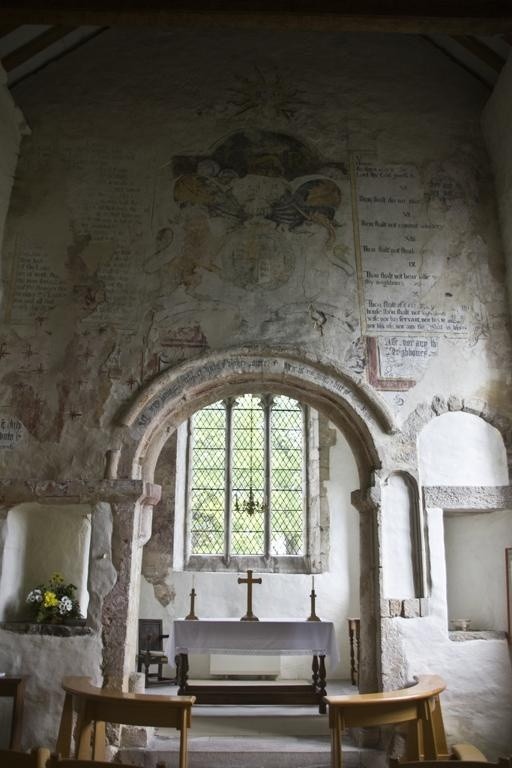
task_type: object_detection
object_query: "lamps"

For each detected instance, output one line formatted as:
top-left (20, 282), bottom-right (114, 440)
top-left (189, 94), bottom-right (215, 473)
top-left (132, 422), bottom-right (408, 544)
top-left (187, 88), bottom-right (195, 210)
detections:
top-left (235, 399), bottom-right (267, 517)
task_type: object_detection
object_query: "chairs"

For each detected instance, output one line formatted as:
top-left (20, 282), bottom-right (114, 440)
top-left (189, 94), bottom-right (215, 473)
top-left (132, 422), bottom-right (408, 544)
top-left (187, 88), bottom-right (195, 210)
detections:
top-left (388, 755), bottom-right (512, 768)
top-left (139, 619), bottom-right (178, 688)
top-left (0, 742), bottom-right (166, 768)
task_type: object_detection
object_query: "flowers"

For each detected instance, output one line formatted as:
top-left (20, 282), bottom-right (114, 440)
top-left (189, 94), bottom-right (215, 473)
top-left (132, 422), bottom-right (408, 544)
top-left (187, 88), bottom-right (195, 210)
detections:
top-left (26, 572), bottom-right (84, 623)
